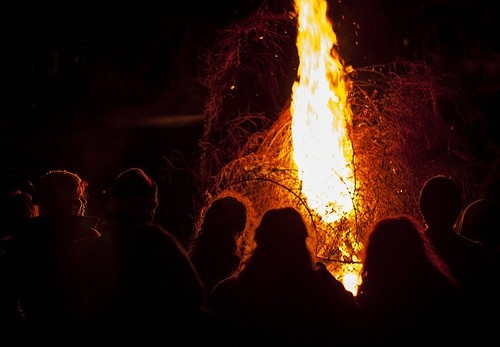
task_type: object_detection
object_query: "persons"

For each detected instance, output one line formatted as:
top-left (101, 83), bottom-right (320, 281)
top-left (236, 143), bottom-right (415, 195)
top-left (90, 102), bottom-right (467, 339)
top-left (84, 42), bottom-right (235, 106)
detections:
top-left (195, 206), bottom-right (358, 347)
top-left (61, 167), bottom-right (210, 346)
top-left (189, 190), bottom-right (251, 292)
top-left (0, 189), bottom-right (39, 249)
top-left (330, 216), bottom-right (485, 347)
top-left (417, 176), bottom-right (490, 347)
top-left (0, 166), bottom-right (107, 347)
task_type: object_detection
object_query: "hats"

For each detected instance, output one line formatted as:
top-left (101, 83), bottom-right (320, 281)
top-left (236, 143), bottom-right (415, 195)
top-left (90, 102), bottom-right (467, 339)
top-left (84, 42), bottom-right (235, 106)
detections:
top-left (108, 168), bottom-right (160, 218)
